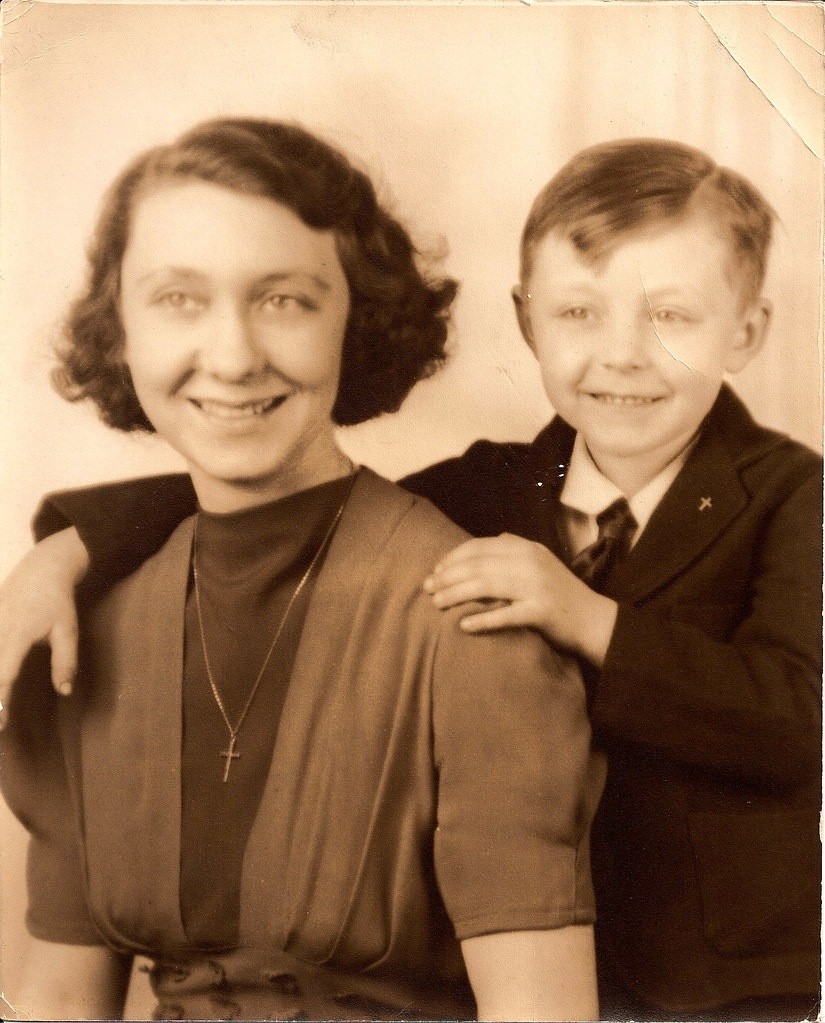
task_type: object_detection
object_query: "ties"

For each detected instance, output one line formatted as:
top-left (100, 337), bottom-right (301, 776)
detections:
top-left (569, 497), bottom-right (639, 593)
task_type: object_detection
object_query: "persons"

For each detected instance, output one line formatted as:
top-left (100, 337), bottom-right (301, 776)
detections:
top-left (1, 142), bottom-right (822, 1023)
top-left (0, 117), bottom-right (609, 1023)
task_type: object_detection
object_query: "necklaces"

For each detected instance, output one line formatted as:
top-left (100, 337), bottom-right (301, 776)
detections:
top-left (193, 462), bottom-right (359, 785)
